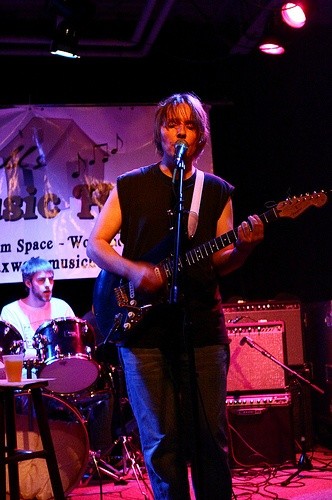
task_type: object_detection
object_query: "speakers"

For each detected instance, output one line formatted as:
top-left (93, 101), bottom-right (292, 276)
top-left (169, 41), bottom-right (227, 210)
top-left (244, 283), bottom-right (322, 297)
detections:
top-left (223, 299), bottom-right (314, 467)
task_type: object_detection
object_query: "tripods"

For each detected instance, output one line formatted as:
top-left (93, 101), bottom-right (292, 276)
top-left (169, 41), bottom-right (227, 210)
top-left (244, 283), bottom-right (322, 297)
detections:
top-left (78, 359), bottom-right (156, 500)
top-left (247, 338), bottom-right (332, 486)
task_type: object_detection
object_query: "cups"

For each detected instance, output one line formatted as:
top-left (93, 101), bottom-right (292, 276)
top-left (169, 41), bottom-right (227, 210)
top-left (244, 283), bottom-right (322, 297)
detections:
top-left (2, 354), bottom-right (23, 382)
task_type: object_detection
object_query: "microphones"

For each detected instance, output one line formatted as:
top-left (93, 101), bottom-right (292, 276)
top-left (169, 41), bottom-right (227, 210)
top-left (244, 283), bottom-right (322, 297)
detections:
top-left (174, 140), bottom-right (188, 167)
top-left (240, 337), bottom-right (249, 345)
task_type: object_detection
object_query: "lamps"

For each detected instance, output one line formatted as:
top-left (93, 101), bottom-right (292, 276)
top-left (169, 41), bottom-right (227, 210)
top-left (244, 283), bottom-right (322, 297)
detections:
top-left (257, 13), bottom-right (293, 56)
top-left (280, 0), bottom-right (323, 31)
top-left (49, 19), bottom-right (81, 60)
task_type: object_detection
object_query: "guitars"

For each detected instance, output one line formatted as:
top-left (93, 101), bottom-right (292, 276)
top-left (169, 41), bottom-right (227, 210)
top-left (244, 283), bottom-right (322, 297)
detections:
top-left (90, 190), bottom-right (329, 344)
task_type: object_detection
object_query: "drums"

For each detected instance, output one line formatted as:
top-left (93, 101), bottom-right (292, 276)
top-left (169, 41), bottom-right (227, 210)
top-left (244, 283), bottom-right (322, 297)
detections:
top-left (6, 389), bottom-right (92, 500)
top-left (61, 345), bottom-right (112, 404)
top-left (32, 316), bottom-right (102, 398)
top-left (0, 319), bottom-right (26, 365)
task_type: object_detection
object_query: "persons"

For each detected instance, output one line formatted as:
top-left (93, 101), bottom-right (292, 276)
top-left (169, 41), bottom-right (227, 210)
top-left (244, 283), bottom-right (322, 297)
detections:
top-left (87, 95), bottom-right (264, 499)
top-left (1, 258), bottom-right (114, 463)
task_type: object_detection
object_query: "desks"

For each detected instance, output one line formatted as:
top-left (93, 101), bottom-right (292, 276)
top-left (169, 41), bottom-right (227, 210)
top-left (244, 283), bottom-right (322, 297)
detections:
top-left (0, 378), bottom-right (66, 500)
